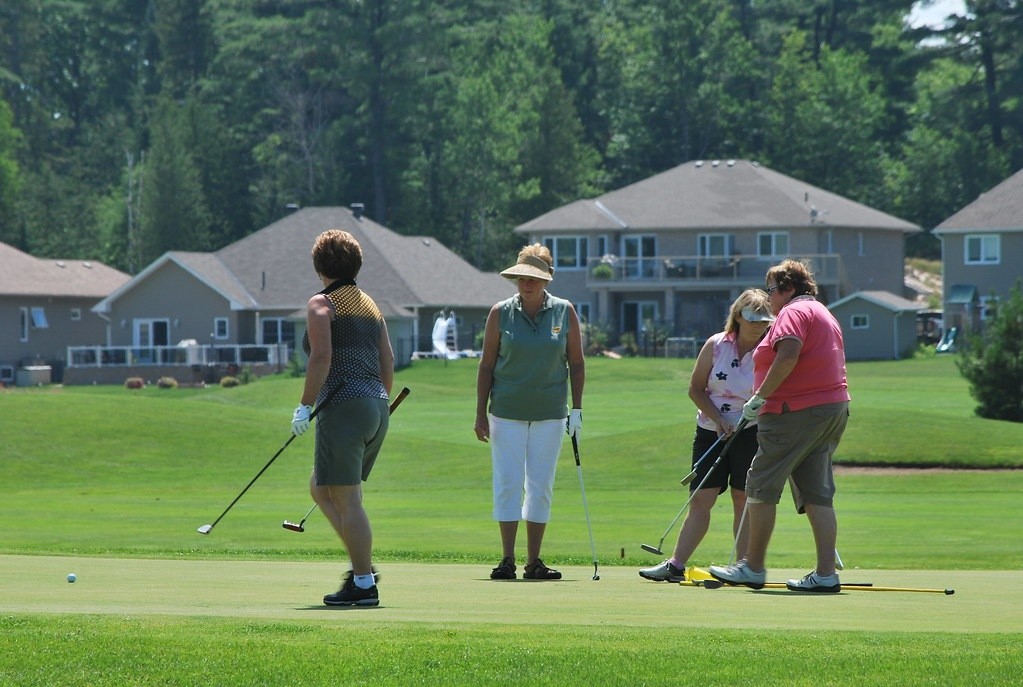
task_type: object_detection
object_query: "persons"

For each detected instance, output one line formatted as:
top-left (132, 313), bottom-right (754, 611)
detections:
top-left (710, 258), bottom-right (850, 592)
top-left (474, 243), bottom-right (586, 580)
top-left (290, 230), bottom-right (394, 606)
top-left (637, 288), bottom-right (776, 582)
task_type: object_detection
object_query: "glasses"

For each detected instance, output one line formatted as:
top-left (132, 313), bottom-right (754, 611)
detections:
top-left (766, 284), bottom-right (780, 292)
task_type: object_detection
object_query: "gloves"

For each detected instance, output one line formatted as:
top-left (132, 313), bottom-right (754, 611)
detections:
top-left (290, 403), bottom-right (312, 436)
top-left (567, 406), bottom-right (584, 447)
top-left (742, 395), bottom-right (766, 420)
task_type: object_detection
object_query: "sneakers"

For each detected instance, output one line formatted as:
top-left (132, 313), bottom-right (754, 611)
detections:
top-left (708, 559), bottom-right (769, 589)
top-left (787, 568), bottom-right (842, 593)
top-left (321, 569), bottom-right (383, 608)
top-left (637, 559), bottom-right (689, 582)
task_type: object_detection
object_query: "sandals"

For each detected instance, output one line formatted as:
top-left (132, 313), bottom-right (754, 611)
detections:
top-left (485, 558), bottom-right (518, 580)
top-left (523, 558), bottom-right (565, 579)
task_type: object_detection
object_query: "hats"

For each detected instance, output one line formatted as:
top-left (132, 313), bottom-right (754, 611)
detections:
top-left (741, 304), bottom-right (780, 324)
top-left (499, 253), bottom-right (557, 282)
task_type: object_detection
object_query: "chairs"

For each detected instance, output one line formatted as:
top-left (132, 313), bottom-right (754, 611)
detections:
top-left (722, 258), bottom-right (741, 276)
top-left (664, 259), bottom-right (686, 277)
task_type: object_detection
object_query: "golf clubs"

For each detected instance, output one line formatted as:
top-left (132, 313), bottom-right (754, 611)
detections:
top-left (834, 548), bottom-right (845, 571)
top-left (704, 499), bottom-right (749, 589)
top-left (679, 415), bottom-right (742, 487)
top-left (566, 413), bottom-right (601, 581)
top-left (641, 406), bottom-right (760, 555)
top-left (195, 377), bottom-right (349, 535)
top-left (280, 386), bottom-right (413, 532)
